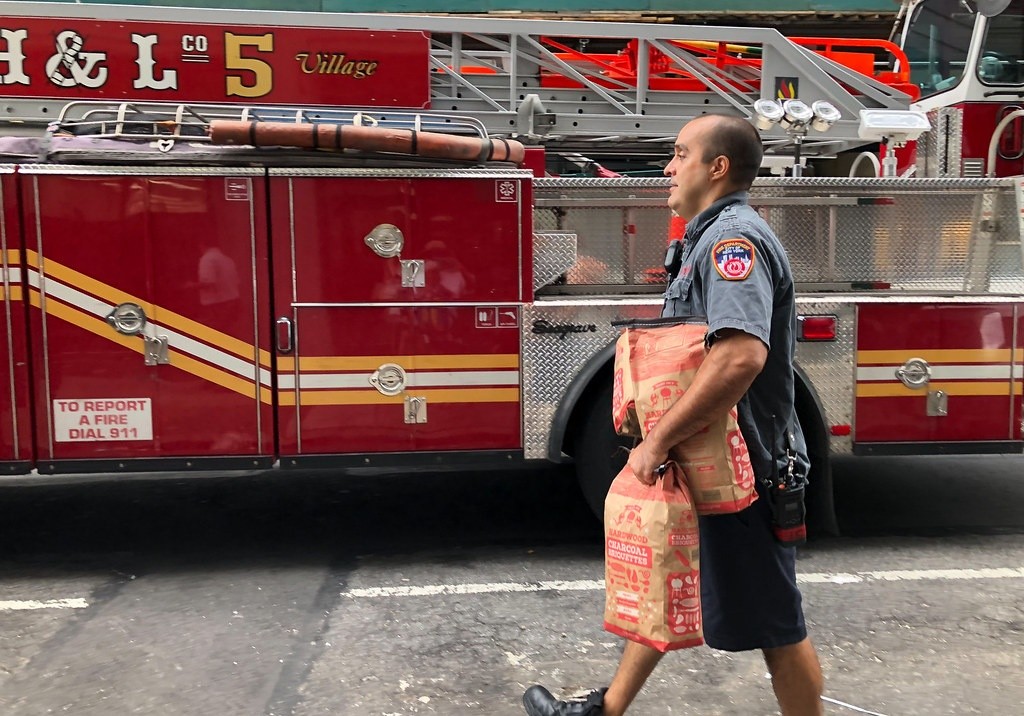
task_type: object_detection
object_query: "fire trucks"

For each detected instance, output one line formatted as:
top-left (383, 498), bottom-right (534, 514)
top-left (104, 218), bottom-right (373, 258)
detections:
top-left (0, 2), bottom-right (1023, 532)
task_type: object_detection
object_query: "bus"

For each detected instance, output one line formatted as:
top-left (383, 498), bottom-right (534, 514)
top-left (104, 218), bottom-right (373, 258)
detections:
top-left (889, 0), bottom-right (1023, 187)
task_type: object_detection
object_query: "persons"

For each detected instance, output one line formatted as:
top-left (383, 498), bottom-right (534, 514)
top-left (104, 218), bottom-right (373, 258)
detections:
top-left (520, 113), bottom-right (830, 715)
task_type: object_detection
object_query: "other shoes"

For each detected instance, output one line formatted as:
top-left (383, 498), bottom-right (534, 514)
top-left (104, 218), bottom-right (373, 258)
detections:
top-left (524, 685), bottom-right (607, 716)
